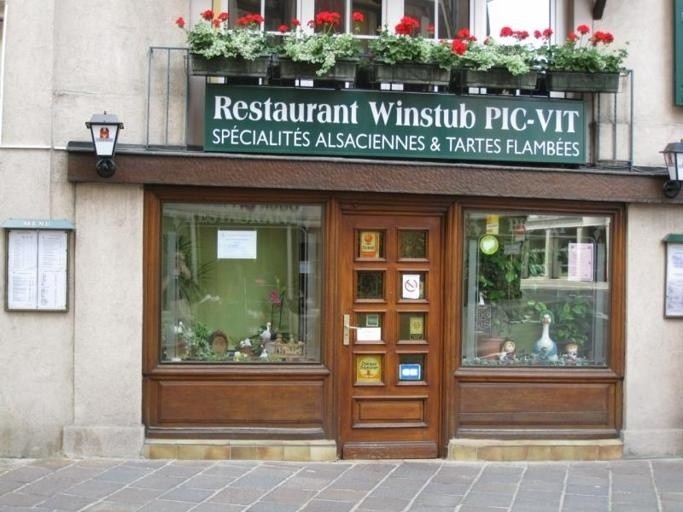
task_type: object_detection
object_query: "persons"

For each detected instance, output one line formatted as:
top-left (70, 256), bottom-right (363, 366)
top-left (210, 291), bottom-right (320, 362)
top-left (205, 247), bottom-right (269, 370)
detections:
top-left (563, 337), bottom-right (580, 364)
top-left (500, 334), bottom-right (517, 364)
top-left (161, 247), bottom-right (192, 319)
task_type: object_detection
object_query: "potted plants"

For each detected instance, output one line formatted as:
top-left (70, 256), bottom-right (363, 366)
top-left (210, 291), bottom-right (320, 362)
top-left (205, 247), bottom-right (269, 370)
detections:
top-left (186, 48), bottom-right (272, 79)
top-left (459, 68), bottom-right (541, 91)
top-left (366, 61), bottom-right (454, 89)
top-left (276, 56), bottom-right (359, 87)
top-left (548, 295), bottom-right (591, 358)
top-left (539, 72), bottom-right (620, 93)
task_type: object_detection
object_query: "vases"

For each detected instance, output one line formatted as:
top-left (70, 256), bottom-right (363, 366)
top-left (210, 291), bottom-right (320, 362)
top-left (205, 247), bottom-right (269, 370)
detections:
top-left (476, 336), bottom-right (505, 360)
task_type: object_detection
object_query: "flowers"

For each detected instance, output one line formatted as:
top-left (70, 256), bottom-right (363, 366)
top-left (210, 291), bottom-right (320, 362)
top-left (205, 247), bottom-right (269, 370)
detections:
top-left (367, 12), bottom-right (459, 69)
top-left (452, 25), bottom-right (546, 79)
top-left (276, 10), bottom-right (364, 78)
top-left (175, 8), bottom-right (268, 60)
top-left (534, 23), bottom-right (632, 76)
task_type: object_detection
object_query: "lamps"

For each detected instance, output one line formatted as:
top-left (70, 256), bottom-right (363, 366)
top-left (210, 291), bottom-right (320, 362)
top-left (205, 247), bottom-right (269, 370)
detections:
top-left (83, 113), bottom-right (124, 178)
top-left (658, 140), bottom-right (682, 200)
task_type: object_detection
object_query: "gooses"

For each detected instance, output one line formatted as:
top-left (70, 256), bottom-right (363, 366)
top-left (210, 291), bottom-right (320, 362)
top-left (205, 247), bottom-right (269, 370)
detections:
top-left (260, 321), bottom-right (272, 341)
top-left (534, 314), bottom-right (559, 362)
top-left (171, 322), bottom-right (185, 335)
top-left (477, 291), bottom-right (485, 307)
